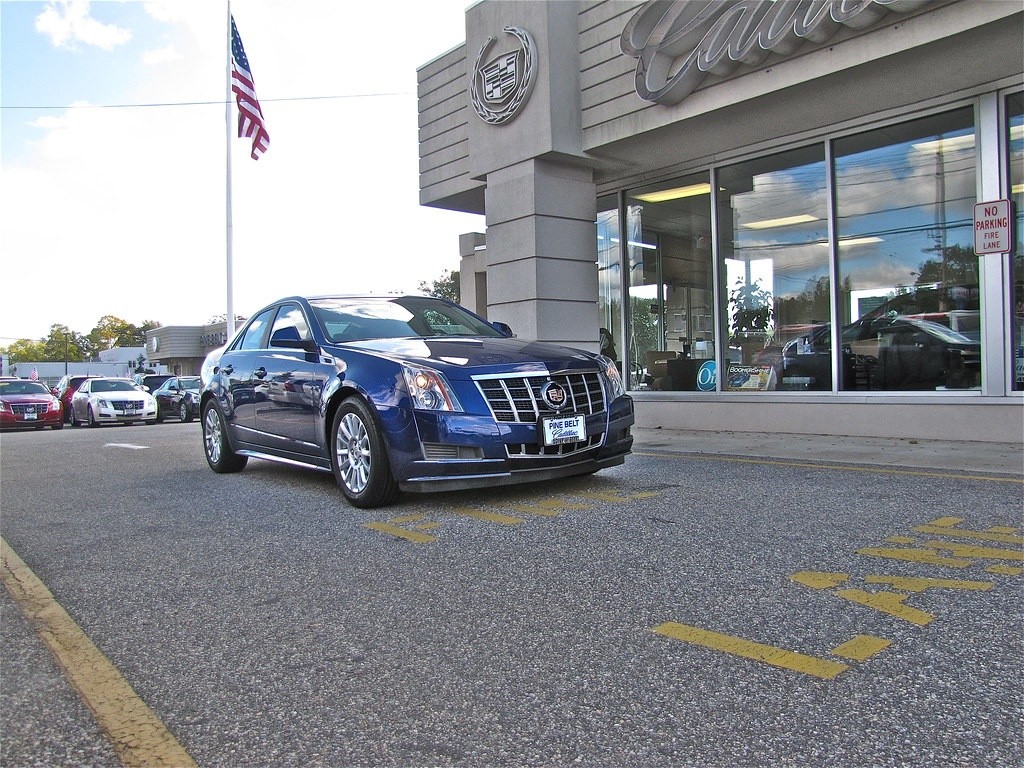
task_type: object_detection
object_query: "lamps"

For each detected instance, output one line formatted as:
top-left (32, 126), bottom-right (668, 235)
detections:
top-left (1011, 184), bottom-right (1024, 194)
top-left (629, 183), bottom-right (726, 204)
top-left (740, 214), bottom-right (821, 230)
top-left (1009, 125), bottom-right (1024, 140)
top-left (911, 133), bottom-right (976, 155)
top-left (816, 236), bottom-right (884, 248)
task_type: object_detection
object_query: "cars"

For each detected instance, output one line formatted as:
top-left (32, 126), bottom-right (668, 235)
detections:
top-left (133, 373), bottom-right (176, 391)
top-left (152, 375), bottom-right (202, 422)
top-left (761, 281), bottom-right (976, 391)
top-left (215, 365), bottom-right (326, 415)
top-left (68, 377), bottom-right (157, 427)
top-left (197, 293), bottom-right (634, 513)
top-left (0, 379), bottom-right (62, 430)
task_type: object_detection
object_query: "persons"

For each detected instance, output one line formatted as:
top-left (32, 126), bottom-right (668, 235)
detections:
top-left (600, 326), bottom-right (617, 368)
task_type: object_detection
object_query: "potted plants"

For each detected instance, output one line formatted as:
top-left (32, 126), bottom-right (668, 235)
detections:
top-left (727, 276), bottom-right (776, 367)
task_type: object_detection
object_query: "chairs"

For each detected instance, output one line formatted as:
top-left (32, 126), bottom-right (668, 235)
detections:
top-left (645, 350), bottom-right (676, 378)
top-left (600, 328), bottom-right (643, 384)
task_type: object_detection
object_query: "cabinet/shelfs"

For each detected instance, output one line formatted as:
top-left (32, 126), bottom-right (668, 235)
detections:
top-left (666, 359), bottom-right (730, 390)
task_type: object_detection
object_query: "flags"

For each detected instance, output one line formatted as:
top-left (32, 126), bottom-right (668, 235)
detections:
top-left (227, 7), bottom-right (272, 162)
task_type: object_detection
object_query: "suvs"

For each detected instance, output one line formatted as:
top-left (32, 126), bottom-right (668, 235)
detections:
top-left (54, 373), bottom-right (105, 424)
